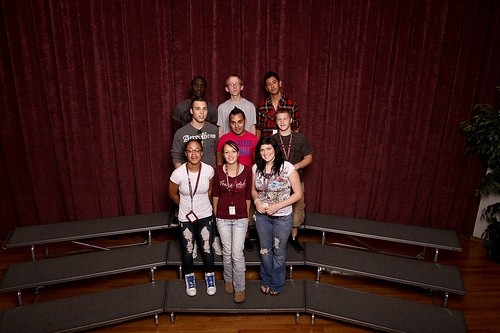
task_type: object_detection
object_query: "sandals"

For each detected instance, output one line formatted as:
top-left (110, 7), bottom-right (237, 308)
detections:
top-left (260, 284), bottom-right (269, 294)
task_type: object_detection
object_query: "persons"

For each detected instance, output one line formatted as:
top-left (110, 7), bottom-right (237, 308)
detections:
top-left (216, 106), bottom-right (259, 252)
top-left (272, 109), bottom-right (312, 253)
top-left (218, 73), bottom-right (257, 139)
top-left (171, 75), bottom-right (218, 135)
top-left (252, 136), bottom-right (302, 296)
top-left (169, 141), bottom-right (217, 296)
top-left (171, 98), bottom-right (223, 259)
top-left (213, 140), bottom-right (253, 302)
top-left (256, 71), bottom-right (299, 139)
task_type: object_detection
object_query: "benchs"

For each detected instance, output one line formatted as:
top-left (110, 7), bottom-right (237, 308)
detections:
top-left (0, 211), bottom-right (466, 333)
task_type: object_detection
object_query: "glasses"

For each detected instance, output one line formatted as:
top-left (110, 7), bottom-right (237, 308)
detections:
top-left (226, 82), bottom-right (243, 87)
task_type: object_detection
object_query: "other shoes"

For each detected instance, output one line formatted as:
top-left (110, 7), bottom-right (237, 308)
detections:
top-left (245, 238), bottom-right (254, 252)
top-left (171, 217), bottom-right (179, 227)
top-left (234, 288), bottom-right (245, 302)
top-left (225, 282), bottom-right (234, 293)
top-left (270, 289), bottom-right (279, 294)
top-left (288, 235), bottom-right (305, 254)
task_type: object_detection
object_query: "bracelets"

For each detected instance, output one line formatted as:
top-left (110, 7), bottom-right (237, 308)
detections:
top-left (254, 199), bottom-right (260, 204)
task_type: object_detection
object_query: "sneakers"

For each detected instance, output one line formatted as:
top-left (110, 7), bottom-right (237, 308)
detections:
top-left (212, 236), bottom-right (223, 255)
top-left (192, 240), bottom-right (199, 259)
top-left (205, 272), bottom-right (217, 296)
top-left (185, 272), bottom-right (197, 297)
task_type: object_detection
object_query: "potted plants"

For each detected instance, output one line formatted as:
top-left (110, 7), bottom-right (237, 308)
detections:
top-left (460, 104), bottom-right (500, 263)
